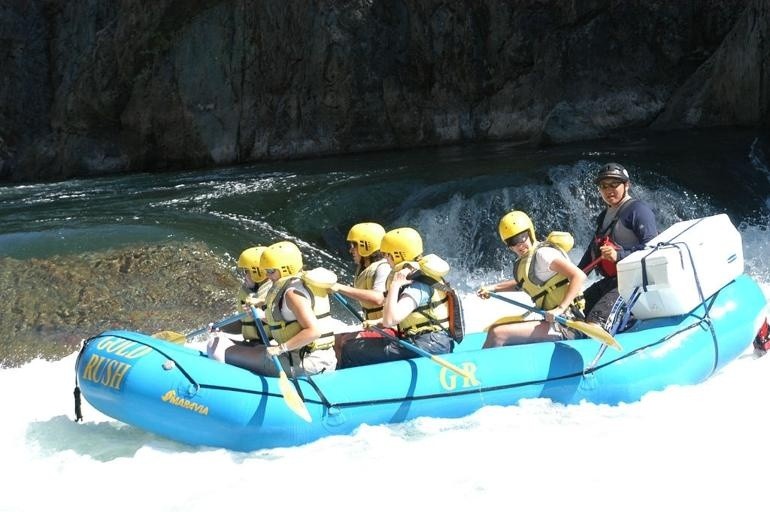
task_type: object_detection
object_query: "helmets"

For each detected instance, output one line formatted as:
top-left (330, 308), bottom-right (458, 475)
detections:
top-left (379, 226), bottom-right (422, 264)
top-left (347, 222), bottom-right (386, 257)
top-left (499, 211), bottom-right (537, 247)
top-left (261, 241), bottom-right (303, 279)
top-left (238, 245), bottom-right (269, 283)
top-left (595, 162), bottom-right (629, 185)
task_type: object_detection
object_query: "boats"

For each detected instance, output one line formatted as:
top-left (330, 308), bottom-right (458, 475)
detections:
top-left (71, 273), bottom-right (770, 453)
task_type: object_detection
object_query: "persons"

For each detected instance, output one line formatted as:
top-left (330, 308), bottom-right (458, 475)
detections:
top-left (329, 222), bottom-right (392, 364)
top-left (478, 211), bottom-right (588, 348)
top-left (576, 162), bottom-right (657, 334)
top-left (206, 247), bottom-right (274, 363)
top-left (340, 227), bottom-right (465, 368)
top-left (224, 242), bottom-right (337, 379)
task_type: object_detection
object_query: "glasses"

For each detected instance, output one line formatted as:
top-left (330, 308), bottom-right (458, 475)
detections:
top-left (351, 242), bottom-right (357, 248)
top-left (383, 253), bottom-right (389, 257)
top-left (266, 269), bottom-right (276, 272)
top-left (598, 182), bottom-right (623, 188)
top-left (242, 269), bottom-right (249, 273)
top-left (505, 230), bottom-right (529, 248)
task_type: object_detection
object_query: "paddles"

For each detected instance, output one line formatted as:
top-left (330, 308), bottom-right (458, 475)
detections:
top-left (478, 290), bottom-right (623, 352)
top-left (240, 299), bottom-right (313, 423)
top-left (150, 311), bottom-right (248, 347)
top-left (362, 323), bottom-right (471, 379)
